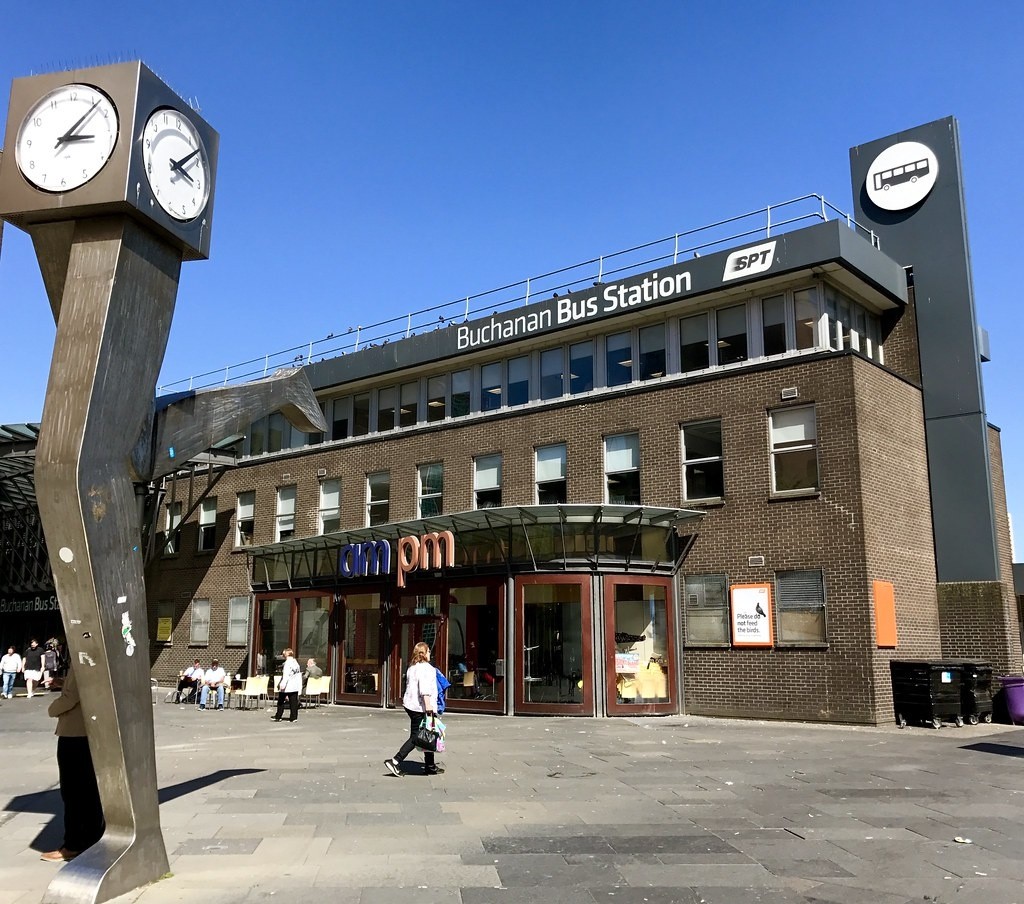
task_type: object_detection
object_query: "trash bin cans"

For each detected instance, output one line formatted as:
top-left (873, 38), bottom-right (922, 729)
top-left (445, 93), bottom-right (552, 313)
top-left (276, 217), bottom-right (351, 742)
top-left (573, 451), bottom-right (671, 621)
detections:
top-left (997, 675), bottom-right (1024, 726)
top-left (888, 655), bottom-right (994, 727)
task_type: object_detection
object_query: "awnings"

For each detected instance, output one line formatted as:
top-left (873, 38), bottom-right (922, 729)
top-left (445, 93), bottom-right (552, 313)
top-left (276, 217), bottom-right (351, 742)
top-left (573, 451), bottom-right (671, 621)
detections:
top-left (229, 504), bottom-right (709, 556)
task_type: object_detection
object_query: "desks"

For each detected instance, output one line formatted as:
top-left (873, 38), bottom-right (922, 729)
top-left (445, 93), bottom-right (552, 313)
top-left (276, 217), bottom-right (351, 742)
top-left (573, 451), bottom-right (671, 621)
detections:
top-left (164, 675), bottom-right (188, 703)
top-left (232, 679), bottom-right (246, 708)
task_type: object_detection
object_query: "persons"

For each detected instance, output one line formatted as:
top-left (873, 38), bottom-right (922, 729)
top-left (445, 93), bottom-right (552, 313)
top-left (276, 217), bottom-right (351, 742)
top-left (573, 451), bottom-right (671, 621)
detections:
top-left (256, 647), bottom-right (267, 675)
top-left (39, 660), bottom-right (106, 862)
top-left (284, 658), bottom-right (322, 709)
top-left (270, 647), bottom-right (300, 722)
top-left (0, 646), bottom-right (22, 699)
top-left (174, 659), bottom-right (205, 704)
top-left (44, 646), bottom-right (57, 689)
top-left (384, 641), bottom-right (444, 778)
top-left (465, 639), bottom-right (478, 665)
top-left (198, 658), bottom-right (225, 711)
top-left (22, 639), bottom-right (46, 698)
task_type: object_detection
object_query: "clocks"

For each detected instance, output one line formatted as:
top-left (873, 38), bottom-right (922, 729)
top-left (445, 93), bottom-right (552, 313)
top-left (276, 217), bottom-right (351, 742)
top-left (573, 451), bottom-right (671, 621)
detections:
top-left (14, 83), bottom-right (120, 194)
top-left (142, 105), bottom-right (212, 224)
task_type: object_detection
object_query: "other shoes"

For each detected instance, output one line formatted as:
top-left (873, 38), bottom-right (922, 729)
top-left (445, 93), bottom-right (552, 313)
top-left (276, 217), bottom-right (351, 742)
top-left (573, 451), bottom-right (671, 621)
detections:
top-left (182, 698), bottom-right (186, 703)
top-left (287, 718), bottom-right (297, 723)
top-left (1, 693), bottom-right (8, 699)
top-left (197, 704), bottom-right (205, 711)
top-left (175, 700), bottom-right (180, 703)
top-left (271, 716), bottom-right (282, 721)
top-left (8, 694), bottom-right (12, 698)
top-left (27, 694), bottom-right (33, 698)
top-left (218, 705), bottom-right (223, 711)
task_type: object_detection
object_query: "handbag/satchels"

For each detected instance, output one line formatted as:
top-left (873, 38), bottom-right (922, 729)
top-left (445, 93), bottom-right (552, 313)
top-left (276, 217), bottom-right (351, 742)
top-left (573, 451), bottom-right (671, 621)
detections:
top-left (413, 711), bottom-right (446, 753)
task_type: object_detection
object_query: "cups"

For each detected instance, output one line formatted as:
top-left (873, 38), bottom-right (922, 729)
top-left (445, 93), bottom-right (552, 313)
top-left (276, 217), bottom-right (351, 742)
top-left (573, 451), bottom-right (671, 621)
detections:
top-left (179, 671), bottom-right (183, 675)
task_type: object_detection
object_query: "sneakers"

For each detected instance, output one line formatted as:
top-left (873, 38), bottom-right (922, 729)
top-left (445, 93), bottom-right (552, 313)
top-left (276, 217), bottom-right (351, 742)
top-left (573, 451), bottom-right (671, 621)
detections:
top-left (40, 845), bottom-right (80, 862)
top-left (384, 759), bottom-right (404, 778)
top-left (424, 763), bottom-right (444, 773)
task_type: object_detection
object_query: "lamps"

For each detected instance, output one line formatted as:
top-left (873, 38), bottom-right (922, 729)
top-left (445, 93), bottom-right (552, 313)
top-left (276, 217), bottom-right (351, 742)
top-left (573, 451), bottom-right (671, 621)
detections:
top-left (832, 331), bottom-right (851, 342)
top-left (391, 409), bottom-right (411, 414)
top-left (619, 348), bottom-right (642, 367)
top-left (805, 321), bottom-right (833, 328)
top-left (488, 386), bottom-right (502, 394)
top-left (705, 341), bottom-right (730, 348)
top-left (561, 360), bottom-right (580, 379)
top-left (427, 401), bottom-right (445, 408)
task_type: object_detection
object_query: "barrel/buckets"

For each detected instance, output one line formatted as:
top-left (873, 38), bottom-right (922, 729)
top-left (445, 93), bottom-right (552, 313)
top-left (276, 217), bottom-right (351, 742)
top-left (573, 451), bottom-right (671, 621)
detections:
top-left (998, 676), bottom-right (1024, 725)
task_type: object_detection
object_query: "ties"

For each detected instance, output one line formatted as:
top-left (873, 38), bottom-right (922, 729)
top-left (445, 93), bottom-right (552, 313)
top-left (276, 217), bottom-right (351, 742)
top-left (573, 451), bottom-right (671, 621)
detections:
top-left (190, 670), bottom-right (195, 676)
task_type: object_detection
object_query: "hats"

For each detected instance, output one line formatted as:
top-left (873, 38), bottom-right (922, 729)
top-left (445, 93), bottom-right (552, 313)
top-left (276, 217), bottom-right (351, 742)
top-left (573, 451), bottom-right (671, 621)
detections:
top-left (212, 659), bottom-right (218, 663)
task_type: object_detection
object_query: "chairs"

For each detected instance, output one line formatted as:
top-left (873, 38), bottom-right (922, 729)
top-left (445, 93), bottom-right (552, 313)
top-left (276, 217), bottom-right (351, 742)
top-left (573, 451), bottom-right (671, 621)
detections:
top-left (187, 676), bottom-right (231, 711)
top-left (151, 678), bottom-right (158, 705)
top-left (235, 675), bottom-right (331, 710)
